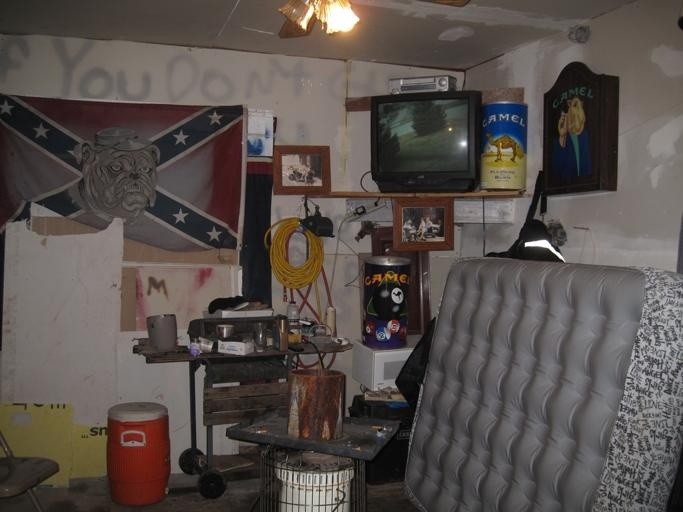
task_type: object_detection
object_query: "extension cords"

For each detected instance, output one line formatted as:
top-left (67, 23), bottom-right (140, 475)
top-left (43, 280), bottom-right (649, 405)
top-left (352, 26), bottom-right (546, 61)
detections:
top-left (344, 198), bottom-right (387, 222)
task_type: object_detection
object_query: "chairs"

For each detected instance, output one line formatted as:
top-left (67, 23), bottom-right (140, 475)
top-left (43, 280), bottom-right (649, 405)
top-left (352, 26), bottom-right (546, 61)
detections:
top-left (0, 433), bottom-right (58, 512)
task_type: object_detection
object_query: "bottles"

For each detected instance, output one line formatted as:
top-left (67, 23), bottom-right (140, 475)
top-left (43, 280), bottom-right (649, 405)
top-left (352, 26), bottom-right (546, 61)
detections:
top-left (327, 305), bottom-right (336, 334)
top-left (287, 301), bottom-right (300, 325)
top-left (189, 336), bottom-right (201, 358)
top-left (255, 322), bottom-right (265, 352)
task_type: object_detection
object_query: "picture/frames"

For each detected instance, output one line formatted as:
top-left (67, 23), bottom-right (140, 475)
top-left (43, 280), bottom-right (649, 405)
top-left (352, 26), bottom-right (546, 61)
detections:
top-left (392, 199), bottom-right (454, 250)
top-left (272, 144), bottom-right (330, 194)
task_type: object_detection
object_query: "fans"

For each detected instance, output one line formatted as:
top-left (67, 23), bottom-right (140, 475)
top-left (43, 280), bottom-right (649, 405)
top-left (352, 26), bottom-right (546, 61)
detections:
top-left (279, 0), bottom-right (471, 38)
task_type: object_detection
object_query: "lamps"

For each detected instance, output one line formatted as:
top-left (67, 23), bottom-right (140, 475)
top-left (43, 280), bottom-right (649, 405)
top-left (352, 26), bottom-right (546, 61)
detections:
top-left (279, 0), bottom-right (360, 34)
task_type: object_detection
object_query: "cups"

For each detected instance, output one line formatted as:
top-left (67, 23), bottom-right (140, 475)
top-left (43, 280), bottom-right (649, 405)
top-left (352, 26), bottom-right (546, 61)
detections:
top-left (216, 324), bottom-right (235, 340)
top-left (146, 314), bottom-right (177, 353)
top-left (198, 335), bottom-right (214, 354)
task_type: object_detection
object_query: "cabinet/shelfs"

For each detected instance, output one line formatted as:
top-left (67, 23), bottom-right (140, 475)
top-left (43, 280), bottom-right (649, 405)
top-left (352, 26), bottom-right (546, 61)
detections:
top-left (329, 87), bottom-right (523, 199)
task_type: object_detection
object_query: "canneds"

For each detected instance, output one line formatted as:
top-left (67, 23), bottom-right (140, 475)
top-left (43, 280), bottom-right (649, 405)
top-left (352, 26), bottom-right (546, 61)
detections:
top-left (363, 255), bottom-right (411, 349)
top-left (480, 101), bottom-right (528, 190)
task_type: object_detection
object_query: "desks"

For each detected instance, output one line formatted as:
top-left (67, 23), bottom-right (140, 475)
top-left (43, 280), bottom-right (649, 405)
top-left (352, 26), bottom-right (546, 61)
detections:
top-left (142, 335), bottom-right (353, 474)
top-left (362, 390), bottom-right (415, 466)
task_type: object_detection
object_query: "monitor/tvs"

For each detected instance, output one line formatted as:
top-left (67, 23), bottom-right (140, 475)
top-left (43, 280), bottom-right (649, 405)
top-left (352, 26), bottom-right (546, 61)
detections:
top-left (370, 91), bottom-right (482, 192)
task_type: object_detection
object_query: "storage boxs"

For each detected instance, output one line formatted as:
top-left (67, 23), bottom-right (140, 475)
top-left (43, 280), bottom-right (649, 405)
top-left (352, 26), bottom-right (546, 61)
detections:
top-left (352, 339), bottom-right (415, 393)
